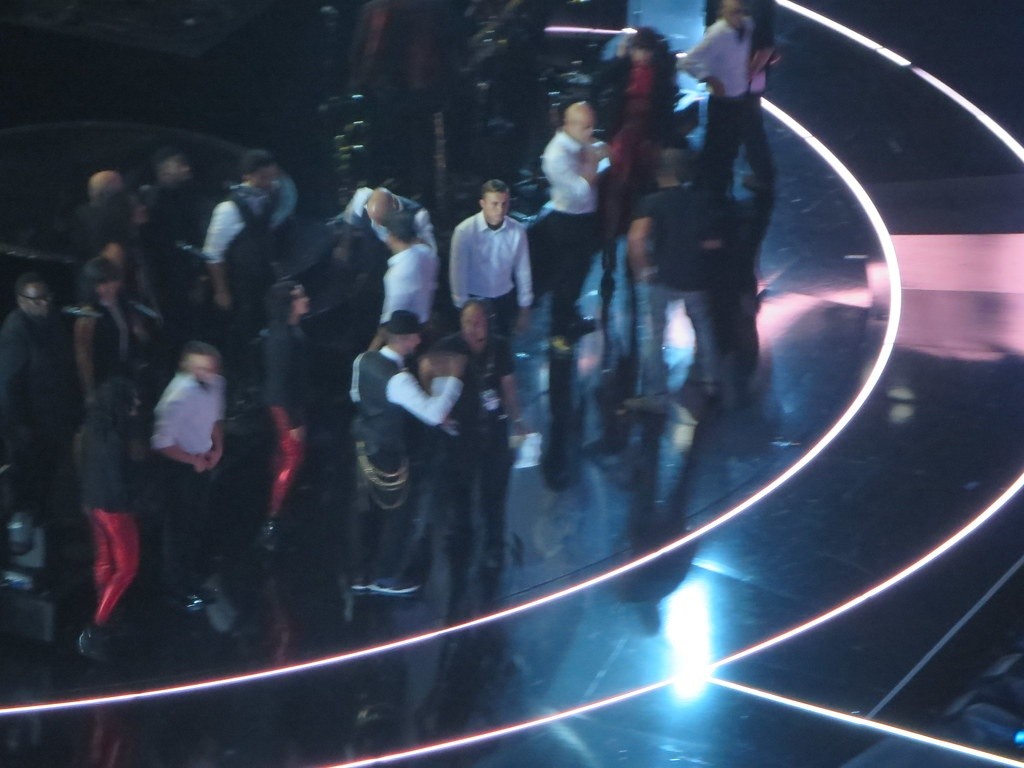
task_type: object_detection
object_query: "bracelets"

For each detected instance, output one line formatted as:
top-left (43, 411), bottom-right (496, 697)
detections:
top-left (639, 266), bottom-right (658, 275)
top-left (512, 415), bottom-right (521, 423)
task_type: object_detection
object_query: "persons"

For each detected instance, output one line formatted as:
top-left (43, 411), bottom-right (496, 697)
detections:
top-left (541, 0), bottom-right (775, 414)
top-left (0, 150), bottom-right (535, 662)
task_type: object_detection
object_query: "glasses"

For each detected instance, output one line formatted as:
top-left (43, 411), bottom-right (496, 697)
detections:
top-left (18, 293), bottom-right (56, 304)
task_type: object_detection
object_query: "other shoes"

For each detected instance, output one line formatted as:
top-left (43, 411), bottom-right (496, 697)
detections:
top-left (75, 622), bottom-right (107, 657)
top-left (372, 578), bottom-right (420, 592)
top-left (332, 568), bottom-right (368, 592)
top-left (176, 583), bottom-right (221, 612)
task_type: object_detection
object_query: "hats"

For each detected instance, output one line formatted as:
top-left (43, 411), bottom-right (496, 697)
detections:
top-left (232, 144), bottom-right (274, 175)
top-left (381, 310), bottom-right (423, 334)
